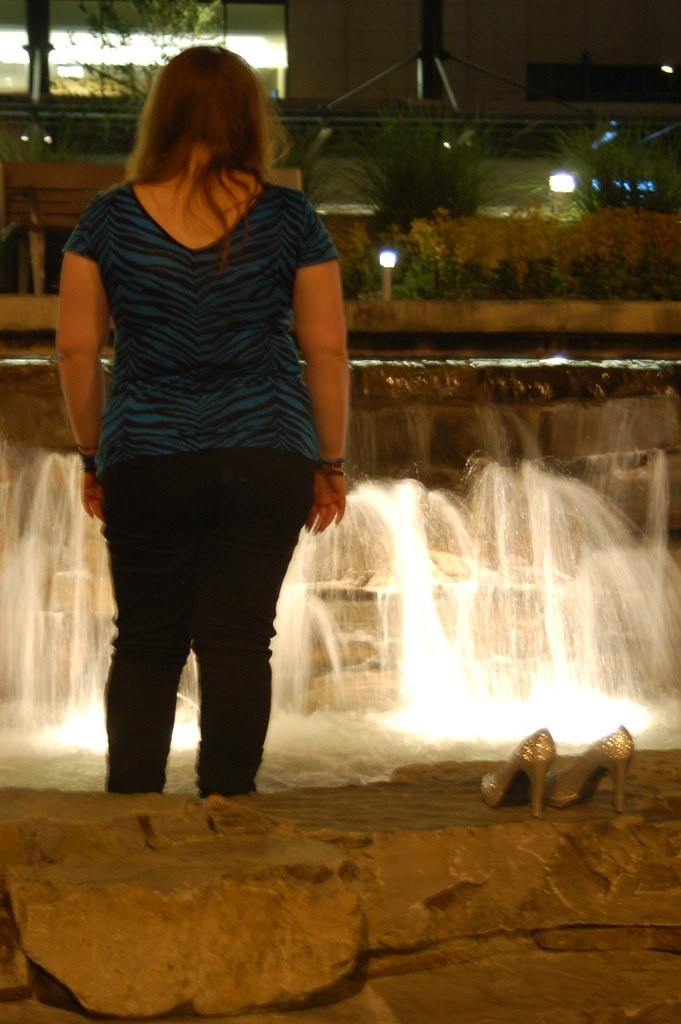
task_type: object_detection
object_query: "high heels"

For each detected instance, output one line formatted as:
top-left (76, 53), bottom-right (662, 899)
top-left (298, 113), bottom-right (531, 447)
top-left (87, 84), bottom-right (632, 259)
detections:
top-left (479, 728), bottom-right (557, 816)
top-left (527, 724), bottom-right (634, 814)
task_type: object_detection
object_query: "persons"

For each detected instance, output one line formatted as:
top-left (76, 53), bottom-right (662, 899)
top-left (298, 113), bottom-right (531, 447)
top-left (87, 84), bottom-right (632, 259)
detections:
top-left (55, 44), bottom-right (350, 797)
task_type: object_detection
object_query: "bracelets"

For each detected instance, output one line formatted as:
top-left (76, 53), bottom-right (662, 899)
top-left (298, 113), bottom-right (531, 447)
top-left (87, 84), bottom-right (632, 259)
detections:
top-left (77, 446), bottom-right (98, 474)
top-left (320, 459), bottom-right (347, 477)
